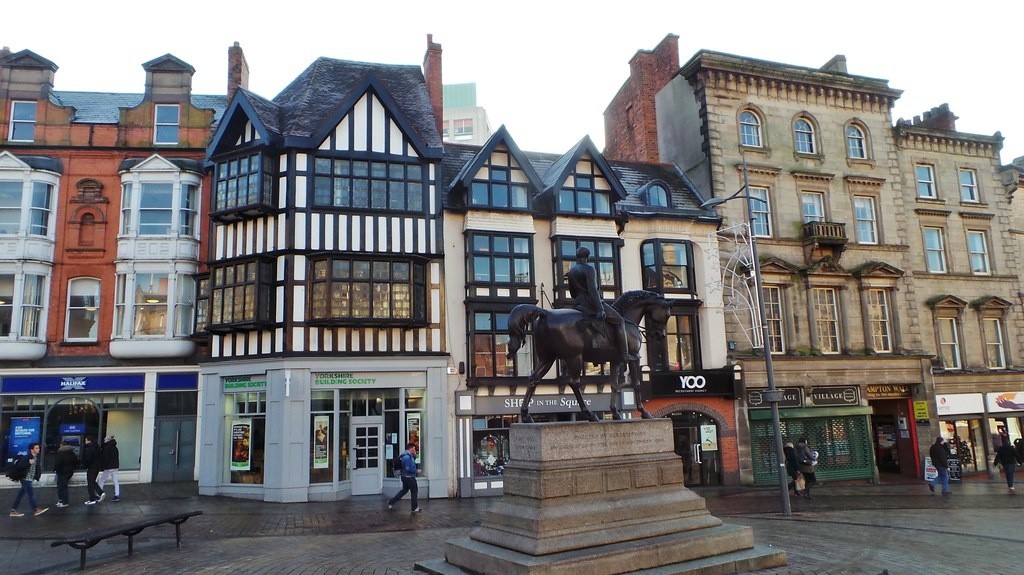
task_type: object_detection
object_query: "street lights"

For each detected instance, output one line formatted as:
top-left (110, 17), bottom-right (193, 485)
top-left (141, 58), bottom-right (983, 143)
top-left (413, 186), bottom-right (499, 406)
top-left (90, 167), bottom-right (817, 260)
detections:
top-left (700, 184), bottom-right (792, 515)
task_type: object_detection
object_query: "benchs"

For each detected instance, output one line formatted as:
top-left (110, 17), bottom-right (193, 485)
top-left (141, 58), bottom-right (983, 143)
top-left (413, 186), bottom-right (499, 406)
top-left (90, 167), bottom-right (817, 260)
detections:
top-left (51, 510), bottom-right (202, 568)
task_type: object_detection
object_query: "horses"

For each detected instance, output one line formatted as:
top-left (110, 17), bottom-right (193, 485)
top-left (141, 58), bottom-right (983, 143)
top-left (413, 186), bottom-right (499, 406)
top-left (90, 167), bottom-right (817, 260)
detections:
top-left (506, 290), bottom-right (676, 423)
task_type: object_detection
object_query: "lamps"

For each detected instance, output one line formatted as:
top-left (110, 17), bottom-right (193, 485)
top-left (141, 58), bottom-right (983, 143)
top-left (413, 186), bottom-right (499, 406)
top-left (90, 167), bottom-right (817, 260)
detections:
top-left (728, 341), bottom-right (735, 350)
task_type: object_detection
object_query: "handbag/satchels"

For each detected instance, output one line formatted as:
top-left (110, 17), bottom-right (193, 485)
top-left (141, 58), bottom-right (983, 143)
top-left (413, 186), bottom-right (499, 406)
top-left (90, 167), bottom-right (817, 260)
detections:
top-left (799, 448), bottom-right (810, 465)
top-left (795, 474), bottom-right (806, 492)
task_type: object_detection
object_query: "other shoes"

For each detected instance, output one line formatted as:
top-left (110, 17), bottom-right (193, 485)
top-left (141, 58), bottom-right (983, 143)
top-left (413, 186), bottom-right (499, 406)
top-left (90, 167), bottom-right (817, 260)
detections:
top-left (929, 483), bottom-right (934, 492)
top-left (794, 492), bottom-right (815, 499)
top-left (56, 500), bottom-right (69, 507)
top-left (1009, 486), bottom-right (1015, 489)
top-left (387, 501), bottom-right (392, 511)
top-left (411, 508), bottom-right (422, 512)
top-left (942, 490), bottom-right (952, 494)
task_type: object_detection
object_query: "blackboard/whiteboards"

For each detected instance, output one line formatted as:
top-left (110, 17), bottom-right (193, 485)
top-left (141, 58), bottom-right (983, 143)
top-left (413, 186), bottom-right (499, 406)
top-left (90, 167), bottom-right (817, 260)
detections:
top-left (947, 459), bottom-right (962, 480)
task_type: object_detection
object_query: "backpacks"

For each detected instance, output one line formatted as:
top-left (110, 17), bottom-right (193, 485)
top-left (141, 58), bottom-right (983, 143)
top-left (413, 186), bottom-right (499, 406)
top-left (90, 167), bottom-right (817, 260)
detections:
top-left (6, 455), bottom-right (32, 481)
top-left (393, 454), bottom-right (411, 477)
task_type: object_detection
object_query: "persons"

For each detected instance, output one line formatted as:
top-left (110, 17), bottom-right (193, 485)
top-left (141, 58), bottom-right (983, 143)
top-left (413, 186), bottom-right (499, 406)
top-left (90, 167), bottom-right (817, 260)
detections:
top-left (993, 437), bottom-right (1022, 491)
top-left (96, 435), bottom-right (120, 502)
top-left (53, 440), bottom-right (77, 508)
top-left (567, 246), bottom-right (638, 362)
top-left (387, 443), bottom-right (423, 512)
top-left (784, 436), bottom-right (819, 499)
top-left (928, 436), bottom-right (952, 494)
top-left (83, 435), bottom-right (107, 505)
top-left (10, 443), bottom-right (49, 516)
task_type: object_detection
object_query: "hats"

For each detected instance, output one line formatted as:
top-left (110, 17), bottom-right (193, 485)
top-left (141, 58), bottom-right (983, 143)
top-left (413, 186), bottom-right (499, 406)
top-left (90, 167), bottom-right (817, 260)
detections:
top-left (104, 435), bottom-right (114, 441)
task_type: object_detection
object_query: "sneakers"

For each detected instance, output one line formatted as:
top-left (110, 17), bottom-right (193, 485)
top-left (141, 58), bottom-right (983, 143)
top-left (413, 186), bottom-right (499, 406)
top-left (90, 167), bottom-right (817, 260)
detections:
top-left (111, 496), bottom-right (120, 501)
top-left (34, 507), bottom-right (49, 515)
top-left (85, 500), bottom-right (96, 505)
top-left (10, 511), bottom-right (25, 516)
top-left (97, 492), bottom-right (106, 503)
